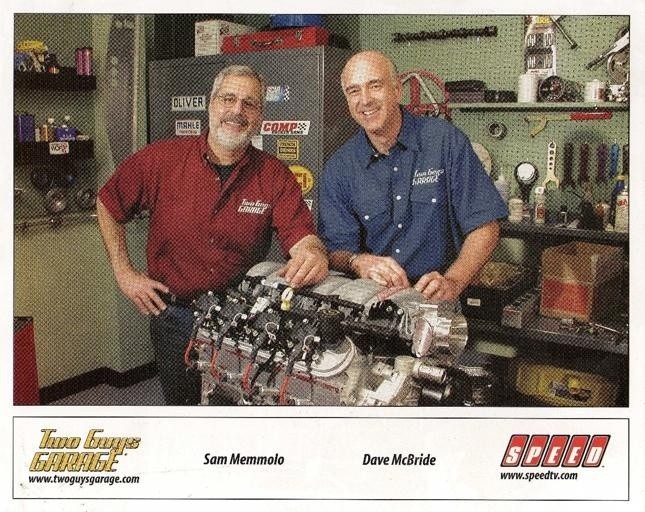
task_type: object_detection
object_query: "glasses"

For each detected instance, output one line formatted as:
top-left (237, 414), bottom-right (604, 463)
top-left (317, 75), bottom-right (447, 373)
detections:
top-left (211, 93), bottom-right (261, 113)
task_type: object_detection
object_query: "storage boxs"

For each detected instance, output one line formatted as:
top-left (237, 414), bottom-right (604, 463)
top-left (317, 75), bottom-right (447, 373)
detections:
top-left (539, 242), bottom-right (623, 319)
top-left (195, 20), bottom-right (257, 56)
top-left (222, 27), bottom-right (330, 54)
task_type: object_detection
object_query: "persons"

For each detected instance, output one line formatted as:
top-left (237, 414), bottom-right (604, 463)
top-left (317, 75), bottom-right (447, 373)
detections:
top-left (318, 51), bottom-right (511, 304)
top-left (95, 64), bottom-right (329, 407)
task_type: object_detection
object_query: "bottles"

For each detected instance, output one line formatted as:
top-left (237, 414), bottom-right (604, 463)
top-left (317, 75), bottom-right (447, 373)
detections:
top-left (76, 45), bottom-right (95, 76)
top-left (610, 175), bottom-right (625, 228)
top-left (614, 186), bottom-right (629, 233)
top-left (494, 176), bottom-right (509, 207)
top-left (533, 193), bottom-right (544, 224)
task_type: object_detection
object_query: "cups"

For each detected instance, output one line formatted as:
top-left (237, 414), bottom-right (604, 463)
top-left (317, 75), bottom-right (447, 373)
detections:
top-left (518, 73), bottom-right (537, 105)
top-left (507, 200), bottom-right (522, 222)
top-left (584, 82), bottom-right (606, 102)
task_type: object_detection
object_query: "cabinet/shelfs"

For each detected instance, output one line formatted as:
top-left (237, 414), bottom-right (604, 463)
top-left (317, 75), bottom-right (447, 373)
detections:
top-left (432, 102), bottom-right (629, 407)
top-left (14, 73), bottom-right (96, 165)
top-left (147, 45), bottom-right (363, 263)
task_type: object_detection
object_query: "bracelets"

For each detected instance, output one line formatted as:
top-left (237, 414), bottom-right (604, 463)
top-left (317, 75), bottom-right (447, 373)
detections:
top-left (348, 252), bottom-right (362, 272)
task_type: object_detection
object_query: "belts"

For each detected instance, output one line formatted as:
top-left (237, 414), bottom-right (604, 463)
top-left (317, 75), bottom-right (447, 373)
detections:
top-left (152, 290), bottom-right (197, 308)
